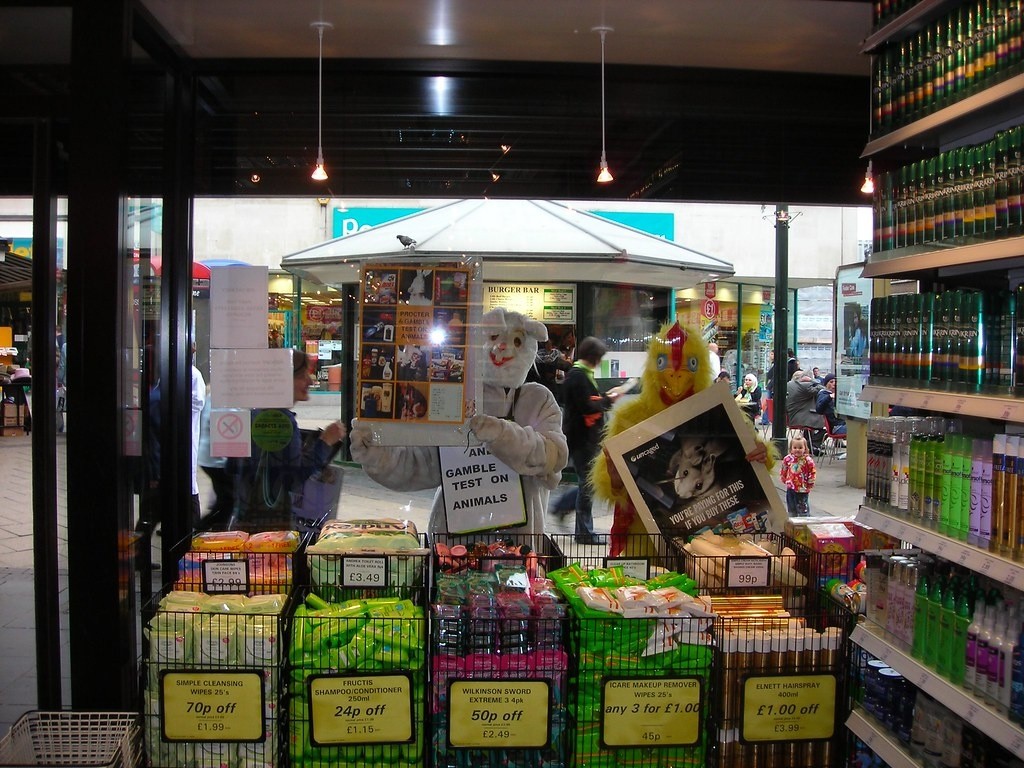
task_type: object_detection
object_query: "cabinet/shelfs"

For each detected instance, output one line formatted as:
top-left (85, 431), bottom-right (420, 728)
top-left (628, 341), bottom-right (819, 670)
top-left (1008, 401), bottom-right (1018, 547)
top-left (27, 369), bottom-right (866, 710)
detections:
top-left (845, 73), bottom-right (1024, 768)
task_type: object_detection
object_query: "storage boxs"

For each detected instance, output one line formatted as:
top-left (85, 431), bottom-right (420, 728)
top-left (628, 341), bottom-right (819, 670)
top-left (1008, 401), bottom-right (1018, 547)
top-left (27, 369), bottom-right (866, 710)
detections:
top-left (1, 404), bottom-right (24, 436)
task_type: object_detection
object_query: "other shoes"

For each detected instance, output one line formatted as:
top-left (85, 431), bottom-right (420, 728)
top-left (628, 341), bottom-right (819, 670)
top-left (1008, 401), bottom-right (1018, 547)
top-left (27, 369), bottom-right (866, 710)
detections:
top-left (554, 505), bottom-right (565, 521)
top-left (583, 537), bottom-right (607, 545)
top-left (759, 423), bottom-right (765, 425)
top-left (834, 440), bottom-right (847, 447)
top-left (808, 442), bottom-right (823, 456)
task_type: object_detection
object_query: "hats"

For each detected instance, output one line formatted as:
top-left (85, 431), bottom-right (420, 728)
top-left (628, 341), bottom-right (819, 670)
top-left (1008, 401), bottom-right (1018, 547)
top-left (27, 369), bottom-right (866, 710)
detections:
top-left (825, 374), bottom-right (835, 385)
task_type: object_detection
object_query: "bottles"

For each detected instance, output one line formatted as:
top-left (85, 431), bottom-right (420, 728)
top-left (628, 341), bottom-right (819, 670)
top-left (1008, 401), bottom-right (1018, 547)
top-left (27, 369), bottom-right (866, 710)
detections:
top-left (863, 659), bottom-right (916, 745)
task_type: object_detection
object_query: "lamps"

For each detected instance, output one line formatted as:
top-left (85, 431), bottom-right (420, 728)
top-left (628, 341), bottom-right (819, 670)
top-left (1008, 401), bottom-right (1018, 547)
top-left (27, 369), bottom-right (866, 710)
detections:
top-left (310, 22), bottom-right (335, 181)
top-left (591, 26), bottom-right (615, 182)
top-left (861, 59), bottom-right (876, 194)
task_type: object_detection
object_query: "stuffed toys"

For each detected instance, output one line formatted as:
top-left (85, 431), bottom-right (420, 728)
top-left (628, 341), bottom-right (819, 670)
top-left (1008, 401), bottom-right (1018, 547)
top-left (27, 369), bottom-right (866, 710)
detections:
top-left (348, 306), bottom-right (570, 556)
top-left (585, 320), bottom-right (780, 573)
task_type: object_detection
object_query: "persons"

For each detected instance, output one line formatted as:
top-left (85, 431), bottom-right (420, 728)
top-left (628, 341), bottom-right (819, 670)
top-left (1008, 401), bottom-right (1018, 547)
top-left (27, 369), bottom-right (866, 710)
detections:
top-left (131, 344), bottom-right (161, 570)
top-left (523, 333), bottom-right (572, 434)
top-left (733, 373), bottom-right (762, 432)
top-left (192, 345), bottom-right (208, 531)
top-left (706, 343), bottom-right (721, 383)
top-left (549, 336), bottom-right (625, 545)
top-left (845, 304), bottom-right (867, 364)
top-left (780, 437), bottom-right (816, 517)
top-left (198, 383), bottom-right (235, 535)
top-left (223, 349), bottom-right (348, 537)
top-left (55, 324), bottom-right (66, 386)
top-left (713, 372), bottom-right (730, 384)
top-left (759, 348), bottom-right (847, 455)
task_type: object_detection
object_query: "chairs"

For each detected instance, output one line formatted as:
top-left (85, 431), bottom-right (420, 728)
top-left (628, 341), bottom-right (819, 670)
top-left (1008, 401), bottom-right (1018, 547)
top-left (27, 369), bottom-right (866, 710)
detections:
top-left (785, 411), bottom-right (814, 459)
top-left (817, 413), bottom-right (848, 465)
top-left (764, 398), bottom-right (773, 439)
